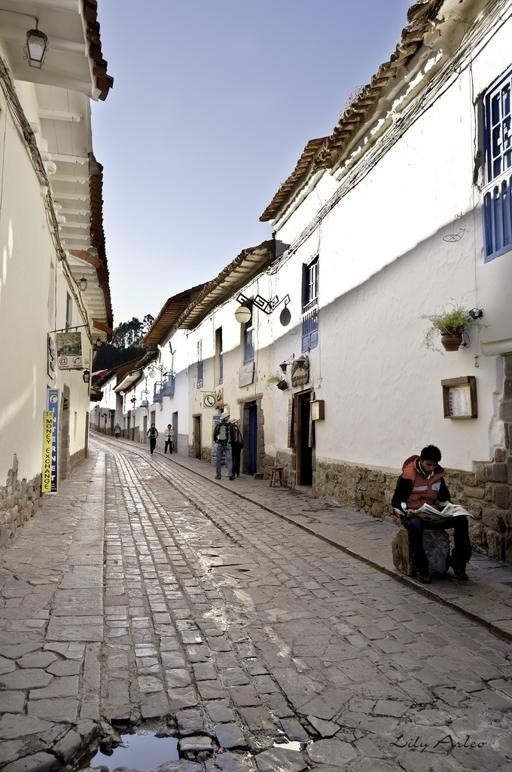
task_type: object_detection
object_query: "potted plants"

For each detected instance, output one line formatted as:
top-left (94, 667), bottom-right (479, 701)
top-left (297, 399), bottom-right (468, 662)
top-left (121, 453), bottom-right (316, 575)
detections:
top-left (419, 298), bottom-right (482, 351)
top-left (266, 374), bottom-right (288, 391)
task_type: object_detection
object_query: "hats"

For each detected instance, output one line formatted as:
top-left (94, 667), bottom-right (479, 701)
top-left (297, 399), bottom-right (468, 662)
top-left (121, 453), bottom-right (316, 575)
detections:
top-left (230, 417), bottom-right (240, 423)
top-left (220, 412), bottom-right (231, 419)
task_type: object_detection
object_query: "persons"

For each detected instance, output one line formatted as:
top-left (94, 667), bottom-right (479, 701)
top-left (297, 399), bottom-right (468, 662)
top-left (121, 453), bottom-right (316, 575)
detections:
top-left (391, 443), bottom-right (473, 583)
top-left (112, 423), bottom-right (121, 438)
top-left (231, 417), bottom-right (244, 478)
top-left (213, 411), bottom-right (235, 481)
top-left (146, 423), bottom-right (158, 455)
top-left (162, 423), bottom-right (174, 454)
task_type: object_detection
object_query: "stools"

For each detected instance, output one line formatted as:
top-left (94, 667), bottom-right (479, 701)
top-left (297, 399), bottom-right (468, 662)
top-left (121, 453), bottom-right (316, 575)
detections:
top-left (270, 467), bottom-right (283, 488)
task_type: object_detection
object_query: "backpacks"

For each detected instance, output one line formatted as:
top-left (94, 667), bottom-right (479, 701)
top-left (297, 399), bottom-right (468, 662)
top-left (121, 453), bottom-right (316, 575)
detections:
top-left (231, 426), bottom-right (245, 448)
top-left (216, 422), bottom-right (228, 443)
top-left (391, 526), bottom-right (414, 577)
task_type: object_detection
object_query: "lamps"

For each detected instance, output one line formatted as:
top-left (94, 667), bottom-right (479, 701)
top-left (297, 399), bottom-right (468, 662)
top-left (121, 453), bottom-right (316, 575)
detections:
top-left (77, 274), bottom-right (88, 291)
top-left (82, 368), bottom-right (91, 383)
top-left (149, 366), bottom-right (163, 378)
top-left (235, 293), bottom-right (271, 324)
top-left (0, 9), bottom-right (46, 69)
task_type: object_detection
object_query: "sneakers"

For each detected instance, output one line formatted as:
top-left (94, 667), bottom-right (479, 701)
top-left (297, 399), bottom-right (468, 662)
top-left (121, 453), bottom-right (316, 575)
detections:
top-left (213, 474), bottom-right (239, 480)
top-left (449, 556), bottom-right (470, 580)
top-left (418, 567), bottom-right (432, 585)
